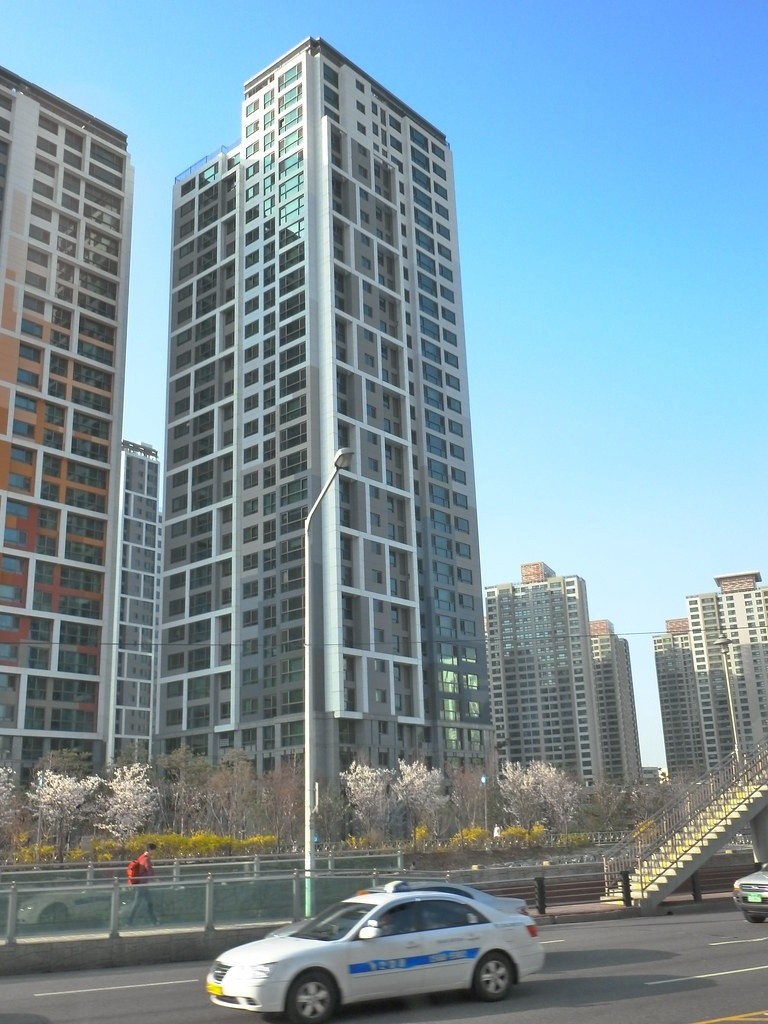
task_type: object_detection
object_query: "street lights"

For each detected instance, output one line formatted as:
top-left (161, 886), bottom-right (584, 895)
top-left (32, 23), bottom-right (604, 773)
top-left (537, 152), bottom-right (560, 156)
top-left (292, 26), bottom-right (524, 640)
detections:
top-left (713, 634), bottom-right (742, 768)
top-left (481, 773), bottom-right (489, 832)
top-left (305, 447), bottom-right (355, 920)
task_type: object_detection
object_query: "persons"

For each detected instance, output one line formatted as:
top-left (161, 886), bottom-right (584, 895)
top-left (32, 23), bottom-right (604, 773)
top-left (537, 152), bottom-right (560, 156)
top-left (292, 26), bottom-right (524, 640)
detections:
top-left (379, 910), bottom-right (402, 935)
top-left (126, 844), bottom-right (160, 930)
top-left (494, 824), bottom-right (505, 839)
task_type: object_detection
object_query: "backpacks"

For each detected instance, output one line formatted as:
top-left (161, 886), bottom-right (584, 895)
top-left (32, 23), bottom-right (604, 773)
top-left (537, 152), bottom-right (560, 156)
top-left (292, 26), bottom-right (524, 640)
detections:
top-left (127, 855), bottom-right (150, 884)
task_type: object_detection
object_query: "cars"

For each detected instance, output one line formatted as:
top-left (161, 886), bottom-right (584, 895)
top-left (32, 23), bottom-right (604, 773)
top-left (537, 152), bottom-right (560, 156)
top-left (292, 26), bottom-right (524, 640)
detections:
top-left (16, 890), bottom-right (137, 928)
top-left (206, 889), bottom-right (544, 1024)
top-left (265, 882), bottom-right (530, 940)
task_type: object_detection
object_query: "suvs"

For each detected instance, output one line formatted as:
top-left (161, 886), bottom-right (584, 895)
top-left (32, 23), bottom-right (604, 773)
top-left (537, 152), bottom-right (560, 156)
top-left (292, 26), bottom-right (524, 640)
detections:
top-left (733, 861), bottom-right (768, 922)
